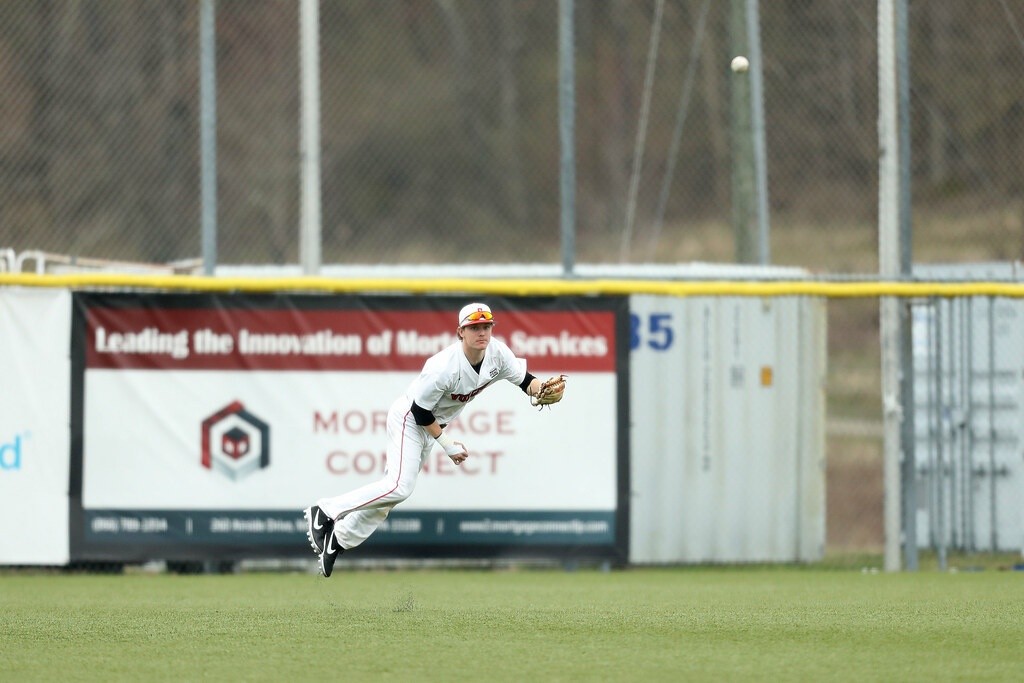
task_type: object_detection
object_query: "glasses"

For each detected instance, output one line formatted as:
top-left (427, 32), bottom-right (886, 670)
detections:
top-left (459, 311), bottom-right (493, 326)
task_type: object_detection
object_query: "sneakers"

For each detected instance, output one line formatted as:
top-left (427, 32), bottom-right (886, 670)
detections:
top-left (318, 530), bottom-right (341, 578)
top-left (304, 506), bottom-right (334, 555)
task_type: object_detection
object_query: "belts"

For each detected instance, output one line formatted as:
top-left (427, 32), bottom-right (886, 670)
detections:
top-left (439, 423), bottom-right (448, 430)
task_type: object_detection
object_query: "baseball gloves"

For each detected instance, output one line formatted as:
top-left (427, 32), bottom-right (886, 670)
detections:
top-left (529, 372), bottom-right (568, 412)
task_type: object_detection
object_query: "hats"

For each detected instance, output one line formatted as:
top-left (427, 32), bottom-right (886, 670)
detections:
top-left (458, 303), bottom-right (494, 327)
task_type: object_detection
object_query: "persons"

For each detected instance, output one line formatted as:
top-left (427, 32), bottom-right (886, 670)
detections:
top-left (302, 303), bottom-right (568, 578)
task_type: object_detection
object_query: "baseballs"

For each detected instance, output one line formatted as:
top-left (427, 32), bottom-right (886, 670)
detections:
top-left (731, 56), bottom-right (750, 75)
